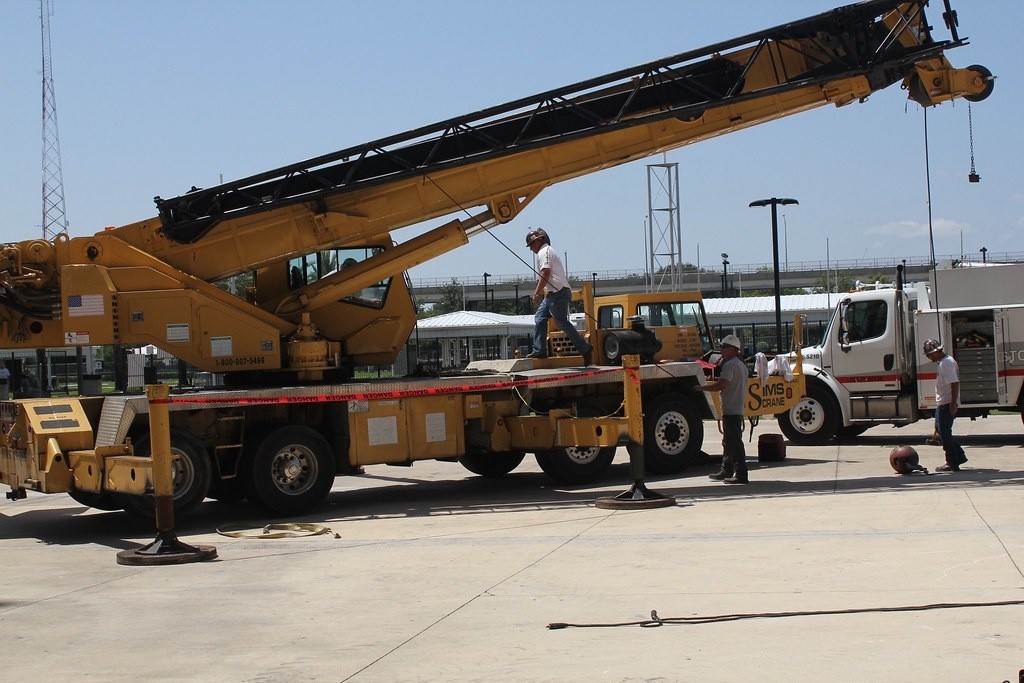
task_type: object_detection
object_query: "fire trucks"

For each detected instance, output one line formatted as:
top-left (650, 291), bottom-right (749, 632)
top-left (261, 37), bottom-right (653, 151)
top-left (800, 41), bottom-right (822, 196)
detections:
top-left (773, 261), bottom-right (1024, 446)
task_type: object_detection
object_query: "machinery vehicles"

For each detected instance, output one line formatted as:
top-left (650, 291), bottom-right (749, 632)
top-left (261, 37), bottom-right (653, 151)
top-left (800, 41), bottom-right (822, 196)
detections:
top-left (0, 0), bottom-right (997, 565)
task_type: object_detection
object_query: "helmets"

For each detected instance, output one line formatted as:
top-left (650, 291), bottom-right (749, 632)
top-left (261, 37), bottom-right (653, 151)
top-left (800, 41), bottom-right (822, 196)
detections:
top-left (923, 339), bottom-right (943, 355)
top-left (526, 230), bottom-right (545, 247)
top-left (722, 335), bottom-right (740, 349)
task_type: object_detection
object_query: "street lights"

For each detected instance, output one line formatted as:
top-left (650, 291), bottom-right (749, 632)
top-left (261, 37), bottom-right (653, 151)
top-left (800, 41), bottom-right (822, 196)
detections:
top-left (749, 197), bottom-right (801, 353)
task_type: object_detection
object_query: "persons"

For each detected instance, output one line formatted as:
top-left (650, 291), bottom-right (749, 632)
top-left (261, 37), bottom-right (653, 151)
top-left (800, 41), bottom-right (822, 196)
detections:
top-left (693, 334), bottom-right (749, 484)
top-left (923, 338), bottom-right (968, 472)
top-left (320, 258), bottom-right (381, 304)
top-left (525, 230), bottom-right (594, 367)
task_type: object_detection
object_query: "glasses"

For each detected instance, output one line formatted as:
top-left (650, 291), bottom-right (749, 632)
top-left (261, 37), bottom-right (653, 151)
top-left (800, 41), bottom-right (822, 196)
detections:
top-left (720, 344), bottom-right (731, 349)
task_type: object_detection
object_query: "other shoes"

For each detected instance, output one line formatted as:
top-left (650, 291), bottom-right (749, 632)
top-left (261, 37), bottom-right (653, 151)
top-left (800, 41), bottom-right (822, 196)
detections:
top-left (722, 476), bottom-right (749, 485)
top-left (936, 462), bottom-right (960, 472)
top-left (526, 352), bottom-right (548, 358)
top-left (709, 471), bottom-right (733, 480)
top-left (945, 454), bottom-right (968, 467)
top-left (582, 344), bottom-right (593, 367)
